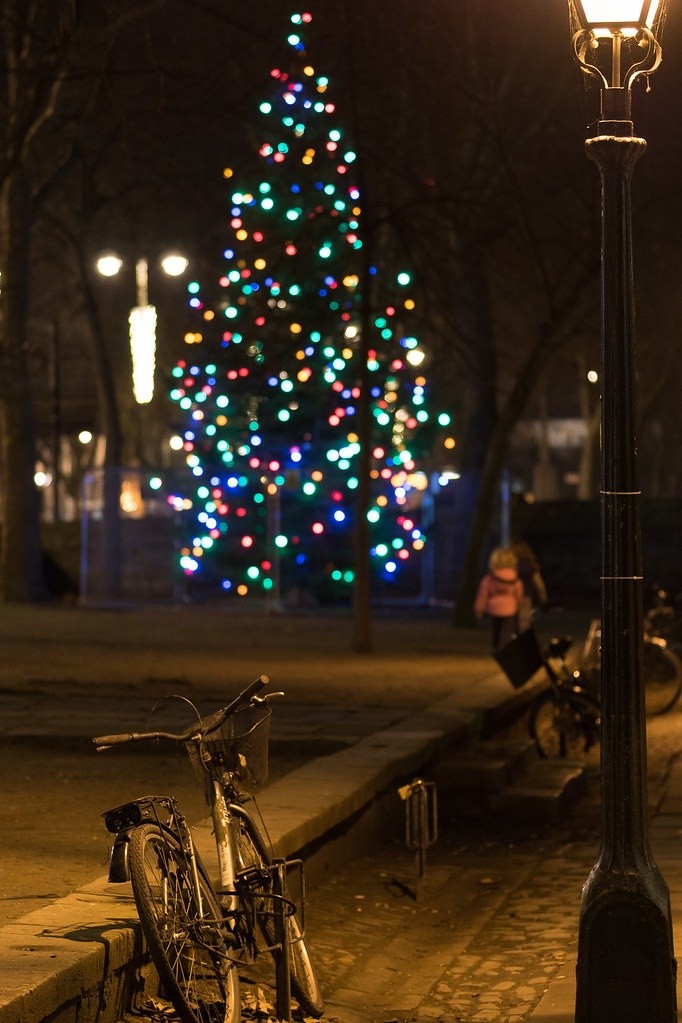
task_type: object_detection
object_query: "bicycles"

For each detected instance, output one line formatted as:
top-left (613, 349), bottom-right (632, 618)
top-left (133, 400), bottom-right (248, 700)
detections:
top-left (629, 587), bottom-right (682, 718)
top-left (493, 608), bottom-right (608, 764)
top-left (92, 675), bottom-right (322, 1023)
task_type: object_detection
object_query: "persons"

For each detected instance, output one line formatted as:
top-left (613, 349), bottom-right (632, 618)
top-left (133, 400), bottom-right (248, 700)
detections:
top-left (473, 532), bottom-right (549, 658)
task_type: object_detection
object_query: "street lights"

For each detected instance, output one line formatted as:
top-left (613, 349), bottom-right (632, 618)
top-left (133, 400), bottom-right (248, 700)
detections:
top-left (562, 0), bottom-right (681, 1023)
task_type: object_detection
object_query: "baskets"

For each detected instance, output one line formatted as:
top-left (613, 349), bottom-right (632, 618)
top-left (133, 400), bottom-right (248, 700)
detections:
top-left (185, 702), bottom-right (269, 789)
